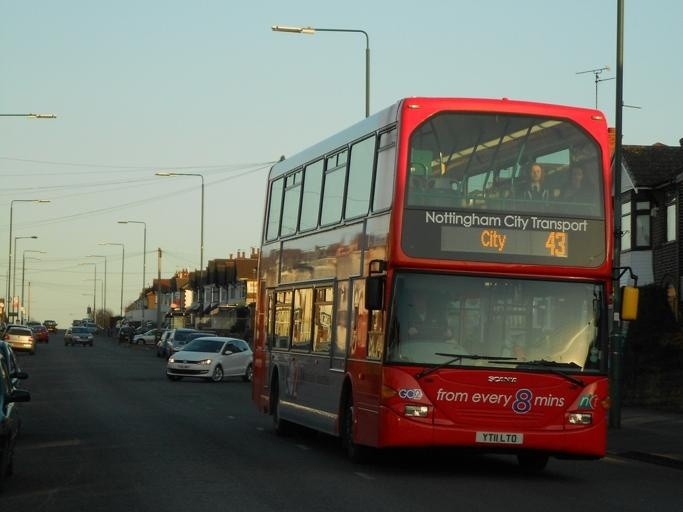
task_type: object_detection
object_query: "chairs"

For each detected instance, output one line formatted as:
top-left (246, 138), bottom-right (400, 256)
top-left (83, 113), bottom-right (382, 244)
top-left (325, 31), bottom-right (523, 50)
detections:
top-left (411, 174), bottom-right (529, 210)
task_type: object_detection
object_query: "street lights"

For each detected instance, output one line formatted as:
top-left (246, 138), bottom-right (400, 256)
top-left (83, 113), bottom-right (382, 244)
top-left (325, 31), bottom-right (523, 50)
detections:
top-left (116, 220), bottom-right (147, 324)
top-left (13, 236), bottom-right (46, 323)
top-left (153, 170), bottom-right (205, 331)
top-left (6, 198), bottom-right (53, 330)
top-left (268, 23), bottom-right (373, 123)
top-left (77, 243), bottom-right (125, 327)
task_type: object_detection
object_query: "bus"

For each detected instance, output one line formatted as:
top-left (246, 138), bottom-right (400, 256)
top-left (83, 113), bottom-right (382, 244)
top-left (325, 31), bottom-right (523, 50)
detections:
top-left (246, 94), bottom-right (640, 462)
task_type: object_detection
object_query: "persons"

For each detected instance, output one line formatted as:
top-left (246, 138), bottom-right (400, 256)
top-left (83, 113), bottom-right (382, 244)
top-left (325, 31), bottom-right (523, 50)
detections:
top-left (561, 167), bottom-right (584, 200)
top-left (395, 288), bottom-right (452, 342)
top-left (517, 163), bottom-right (551, 211)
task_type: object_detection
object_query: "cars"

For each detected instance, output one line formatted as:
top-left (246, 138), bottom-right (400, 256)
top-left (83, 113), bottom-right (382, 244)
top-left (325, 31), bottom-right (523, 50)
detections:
top-left (0, 312), bottom-right (98, 483)
top-left (111, 323), bottom-right (255, 385)
